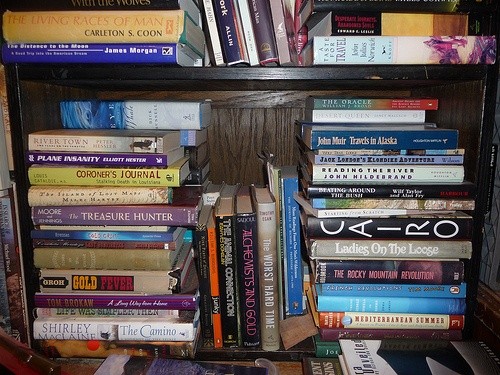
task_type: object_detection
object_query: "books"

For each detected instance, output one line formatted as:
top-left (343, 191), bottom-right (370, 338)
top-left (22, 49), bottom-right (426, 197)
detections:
top-left (0, 0), bottom-right (500, 66)
top-left (0, 94), bottom-right (500, 375)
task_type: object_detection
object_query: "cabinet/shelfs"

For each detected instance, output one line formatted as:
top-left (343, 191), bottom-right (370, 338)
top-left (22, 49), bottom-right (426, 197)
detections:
top-left (0, 0), bottom-right (500, 375)
